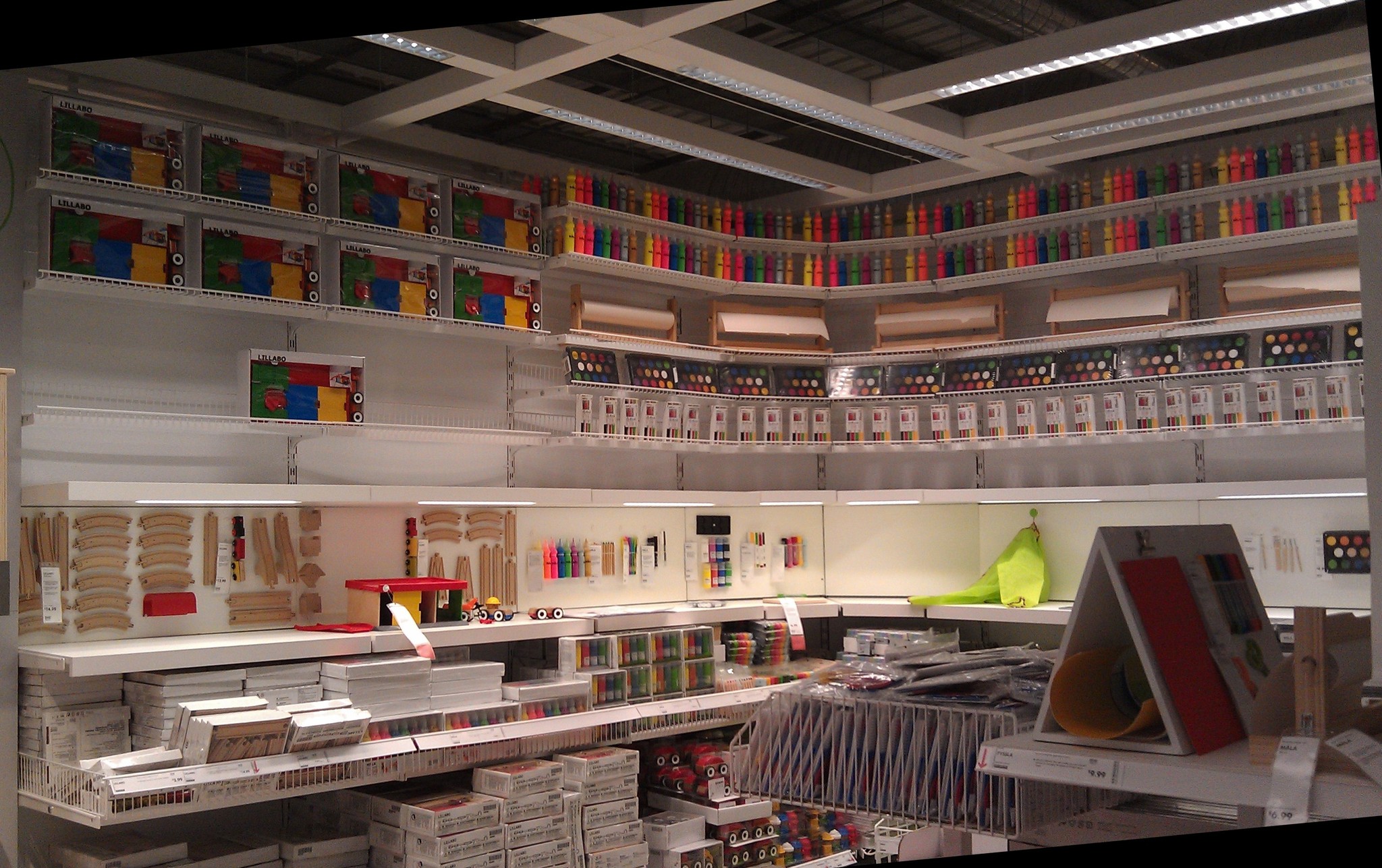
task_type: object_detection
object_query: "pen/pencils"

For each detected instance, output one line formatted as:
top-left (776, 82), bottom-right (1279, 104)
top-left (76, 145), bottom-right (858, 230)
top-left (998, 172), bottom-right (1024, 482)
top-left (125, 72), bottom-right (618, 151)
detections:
top-left (602, 542), bottom-right (615, 576)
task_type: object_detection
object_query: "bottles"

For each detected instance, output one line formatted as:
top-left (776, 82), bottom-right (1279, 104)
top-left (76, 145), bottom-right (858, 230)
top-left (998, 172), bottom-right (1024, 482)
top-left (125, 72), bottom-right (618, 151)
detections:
top-left (519, 120), bottom-right (1374, 286)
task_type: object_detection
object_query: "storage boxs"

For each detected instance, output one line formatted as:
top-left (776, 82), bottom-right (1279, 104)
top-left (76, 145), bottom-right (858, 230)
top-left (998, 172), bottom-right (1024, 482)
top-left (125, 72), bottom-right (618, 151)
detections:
top-left (13, 608), bottom-right (934, 868)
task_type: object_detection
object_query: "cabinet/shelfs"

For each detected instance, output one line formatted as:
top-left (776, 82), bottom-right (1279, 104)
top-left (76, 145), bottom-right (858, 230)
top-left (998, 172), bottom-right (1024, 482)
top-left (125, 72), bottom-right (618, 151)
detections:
top-left (13, 438), bottom-right (840, 868)
top-left (23, 75), bottom-right (548, 349)
top-left (833, 477), bottom-right (1370, 635)
top-left (542, 164), bottom-right (1379, 446)
